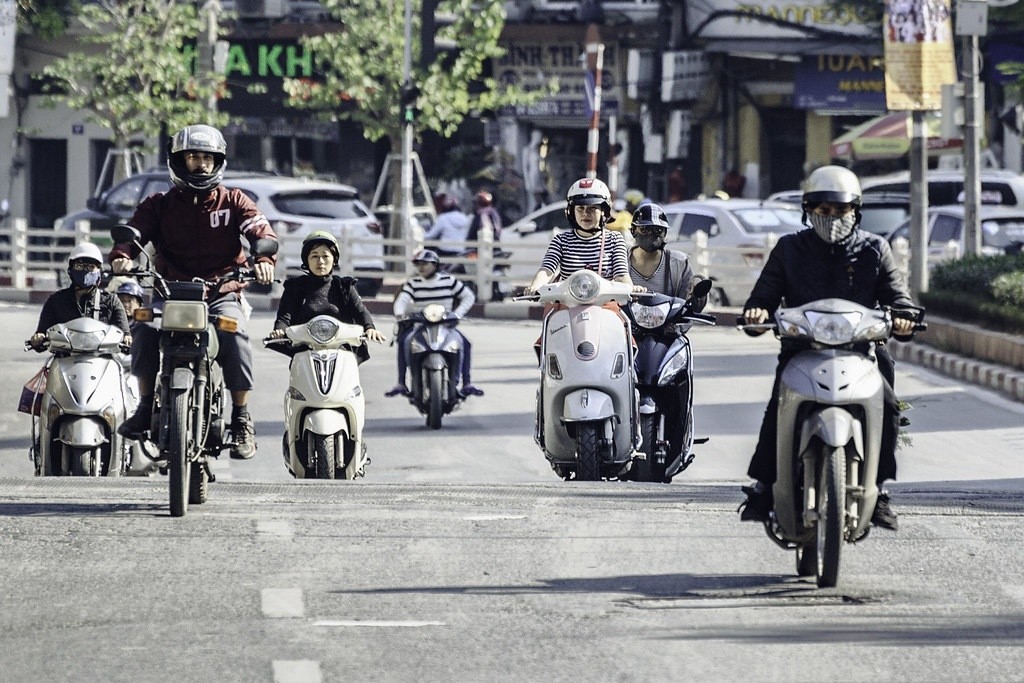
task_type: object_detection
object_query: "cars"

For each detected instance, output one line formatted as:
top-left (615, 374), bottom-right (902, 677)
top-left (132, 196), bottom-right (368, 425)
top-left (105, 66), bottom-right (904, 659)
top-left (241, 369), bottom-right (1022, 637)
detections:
top-left (884, 202), bottom-right (1024, 272)
top-left (373, 205), bottom-right (437, 270)
top-left (446, 199), bottom-right (577, 304)
top-left (661, 189), bottom-right (810, 310)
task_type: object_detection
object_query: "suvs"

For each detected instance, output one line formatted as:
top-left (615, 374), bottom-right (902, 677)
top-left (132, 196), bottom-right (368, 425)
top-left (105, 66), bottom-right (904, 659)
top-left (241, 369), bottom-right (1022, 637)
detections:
top-left (859, 167), bottom-right (1024, 237)
top-left (49, 165), bottom-right (386, 301)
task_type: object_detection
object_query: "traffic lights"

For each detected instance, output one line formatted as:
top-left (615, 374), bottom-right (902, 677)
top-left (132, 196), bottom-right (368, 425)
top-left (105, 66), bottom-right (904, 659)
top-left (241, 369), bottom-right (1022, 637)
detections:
top-left (421, 0), bottom-right (458, 67)
top-left (401, 83), bottom-right (423, 125)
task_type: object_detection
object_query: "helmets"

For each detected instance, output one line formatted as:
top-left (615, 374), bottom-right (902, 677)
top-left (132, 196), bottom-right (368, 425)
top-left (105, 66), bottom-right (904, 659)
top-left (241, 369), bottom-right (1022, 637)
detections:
top-left (631, 203), bottom-right (670, 229)
top-left (801, 164), bottom-right (863, 212)
top-left (68, 241), bottom-right (103, 271)
top-left (301, 231), bottom-right (339, 265)
top-left (114, 282), bottom-right (143, 303)
top-left (411, 250), bottom-right (440, 265)
top-left (167, 124), bottom-right (227, 192)
top-left (566, 178), bottom-right (613, 220)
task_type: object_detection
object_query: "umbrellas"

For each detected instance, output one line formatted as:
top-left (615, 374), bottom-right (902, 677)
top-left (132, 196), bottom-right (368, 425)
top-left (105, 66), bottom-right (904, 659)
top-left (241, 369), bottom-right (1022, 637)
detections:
top-left (829, 111), bottom-right (986, 158)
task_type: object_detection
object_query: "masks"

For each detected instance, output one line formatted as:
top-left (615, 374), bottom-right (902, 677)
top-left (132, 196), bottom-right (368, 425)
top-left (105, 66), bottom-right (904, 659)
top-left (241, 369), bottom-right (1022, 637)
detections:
top-left (69, 268), bottom-right (100, 288)
top-left (809, 207), bottom-right (856, 243)
top-left (633, 230), bottom-right (665, 252)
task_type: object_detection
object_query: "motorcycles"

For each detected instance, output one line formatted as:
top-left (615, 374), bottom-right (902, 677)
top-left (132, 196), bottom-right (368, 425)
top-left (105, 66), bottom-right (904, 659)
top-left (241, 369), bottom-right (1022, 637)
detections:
top-left (513, 274), bottom-right (728, 475)
top-left (393, 304), bottom-right (471, 431)
top-left (735, 296), bottom-right (936, 584)
top-left (261, 315), bottom-right (383, 478)
top-left (108, 223), bottom-right (278, 514)
top-left (0, 199), bottom-right (13, 273)
top-left (23, 318), bottom-right (166, 477)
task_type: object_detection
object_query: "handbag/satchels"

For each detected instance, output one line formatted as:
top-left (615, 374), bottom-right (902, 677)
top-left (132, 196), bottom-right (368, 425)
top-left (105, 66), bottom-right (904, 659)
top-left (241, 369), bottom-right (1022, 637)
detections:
top-left (17, 365), bottom-right (49, 416)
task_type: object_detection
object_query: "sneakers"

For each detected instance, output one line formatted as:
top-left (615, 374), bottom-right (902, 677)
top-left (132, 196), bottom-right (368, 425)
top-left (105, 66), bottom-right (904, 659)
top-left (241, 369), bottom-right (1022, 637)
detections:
top-left (867, 493), bottom-right (898, 532)
top-left (740, 487), bottom-right (774, 522)
top-left (117, 406), bottom-right (157, 440)
top-left (230, 413), bottom-right (255, 459)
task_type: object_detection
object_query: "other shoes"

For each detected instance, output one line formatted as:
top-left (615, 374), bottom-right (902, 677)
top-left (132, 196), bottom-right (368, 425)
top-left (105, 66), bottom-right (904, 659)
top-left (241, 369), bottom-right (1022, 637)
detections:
top-left (385, 383), bottom-right (408, 397)
top-left (460, 386), bottom-right (484, 396)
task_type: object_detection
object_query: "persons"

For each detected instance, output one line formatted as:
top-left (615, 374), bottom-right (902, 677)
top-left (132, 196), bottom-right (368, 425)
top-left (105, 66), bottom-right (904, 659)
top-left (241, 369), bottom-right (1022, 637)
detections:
top-left (628, 202), bottom-right (695, 300)
top-left (424, 191), bottom-right (502, 257)
top-left (886, 0), bottom-right (949, 42)
top-left (384, 250), bottom-right (484, 396)
top-left (523, 130), bottom-right (546, 193)
top-left (271, 231), bottom-right (386, 366)
top-left (526, 179), bottom-right (646, 302)
top-left (31, 242), bottom-right (144, 361)
top-left (742, 166), bottom-right (915, 531)
top-left (109, 123), bottom-right (278, 459)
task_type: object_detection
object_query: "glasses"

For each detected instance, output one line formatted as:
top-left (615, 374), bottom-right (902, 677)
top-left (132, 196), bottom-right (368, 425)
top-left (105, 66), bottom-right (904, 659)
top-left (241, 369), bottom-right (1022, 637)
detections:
top-left (71, 264), bottom-right (101, 271)
top-left (635, 226), bottom-right (665, 234)
